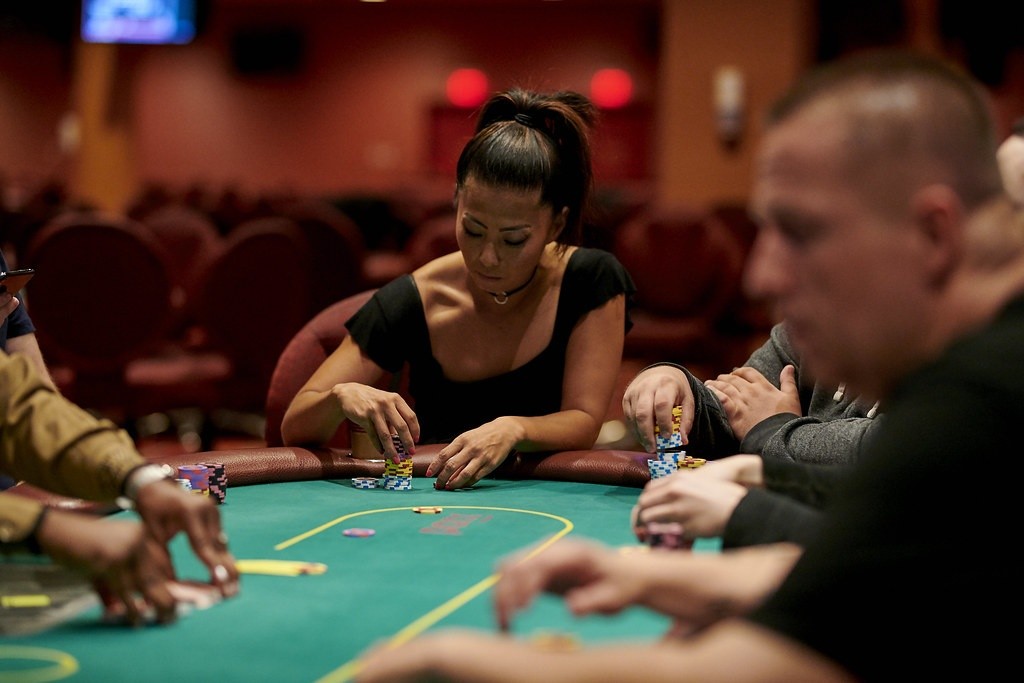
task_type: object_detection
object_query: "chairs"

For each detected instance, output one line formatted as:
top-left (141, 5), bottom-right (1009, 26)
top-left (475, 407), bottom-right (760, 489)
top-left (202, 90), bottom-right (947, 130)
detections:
top-left (0, 167), bottom-right (772, 472)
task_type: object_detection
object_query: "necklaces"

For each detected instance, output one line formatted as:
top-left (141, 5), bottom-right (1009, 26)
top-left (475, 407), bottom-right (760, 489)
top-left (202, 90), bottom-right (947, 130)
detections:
top-left (488, 265), bottom-right (539, 305)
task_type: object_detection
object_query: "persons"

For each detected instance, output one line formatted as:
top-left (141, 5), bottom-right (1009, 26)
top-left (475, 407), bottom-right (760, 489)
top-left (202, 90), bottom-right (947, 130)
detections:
top-left (282, 92), bottom-right (637, 491)
top-left (0, 351), bottom-right (241, 625)
top-left (622, 318), bottom-right (881, 465)
top-left (630, 452), bottom-right (843, 551)
top-left (359, 49), bottom-right (1024, 683)
top-left (0, 250), bottom-right (49, 375)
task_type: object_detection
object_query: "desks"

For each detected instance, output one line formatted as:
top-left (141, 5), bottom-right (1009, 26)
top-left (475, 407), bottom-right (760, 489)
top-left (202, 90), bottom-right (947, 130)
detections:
top-left (0, 445), bottom-right (720, 683)
